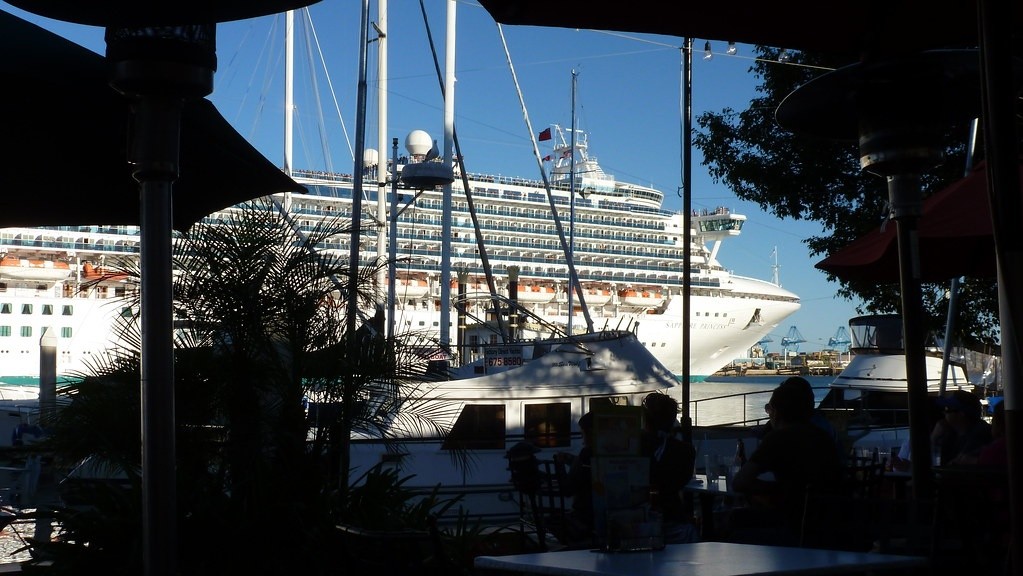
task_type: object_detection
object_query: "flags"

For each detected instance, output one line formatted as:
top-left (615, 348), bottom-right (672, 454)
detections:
top-left (539, 128), bottom-right (551, 141)
top-left (560, 149), bottom-right (572, 160)
top-left (541, 155), bottom-right (550, 163)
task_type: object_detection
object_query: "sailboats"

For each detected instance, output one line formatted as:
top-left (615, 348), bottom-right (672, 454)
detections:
top-left (210, 0), bottom-right (682, 532)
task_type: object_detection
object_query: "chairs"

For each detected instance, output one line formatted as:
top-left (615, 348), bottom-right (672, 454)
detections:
top-left (527, 460), bottom-right (585, 548)
top-left (511, 456), bottom-right (565, 548)
top-left (799, 453), bottom-right (867, 549)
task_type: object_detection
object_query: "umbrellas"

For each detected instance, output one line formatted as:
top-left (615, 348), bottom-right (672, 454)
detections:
top-left (0, 8), bottom-right (310, 239)
top-left (815, 163), bottom-right (999, 290)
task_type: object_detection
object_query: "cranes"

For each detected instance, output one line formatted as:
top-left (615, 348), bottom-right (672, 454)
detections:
top-left (828, 326), bottom-right (852, 346)
top-left (758, 334), bottom-right (773, 344)
top-left (780, 326), bottom-right (807, 346)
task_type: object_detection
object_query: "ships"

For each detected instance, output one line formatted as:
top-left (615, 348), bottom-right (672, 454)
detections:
top-left (6, 122), bottom-right (803, 384)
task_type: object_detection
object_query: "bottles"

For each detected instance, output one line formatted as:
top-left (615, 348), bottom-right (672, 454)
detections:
top-left (868, 447), bottom-right (881, 477)
top-left (732, 438), bottom-right (749, 494)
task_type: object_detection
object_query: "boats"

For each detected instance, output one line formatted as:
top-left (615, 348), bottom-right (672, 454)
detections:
top-left (0, 401), bottom-right (72, 450)
top-left (828, 311), bottom-right (976, 400)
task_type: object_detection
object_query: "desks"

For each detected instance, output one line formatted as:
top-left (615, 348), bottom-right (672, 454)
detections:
top-left (475, 543), bottom-right (925, 575)
top-left (688, 463), bottom-right (739, 543)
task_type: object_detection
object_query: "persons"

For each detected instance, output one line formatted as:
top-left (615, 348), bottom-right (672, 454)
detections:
top-left (532, 375), bottom-right (1022, 557)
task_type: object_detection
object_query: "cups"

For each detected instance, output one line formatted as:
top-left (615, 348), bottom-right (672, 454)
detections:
top-left (705, 449), bottom-right (722, 483)
